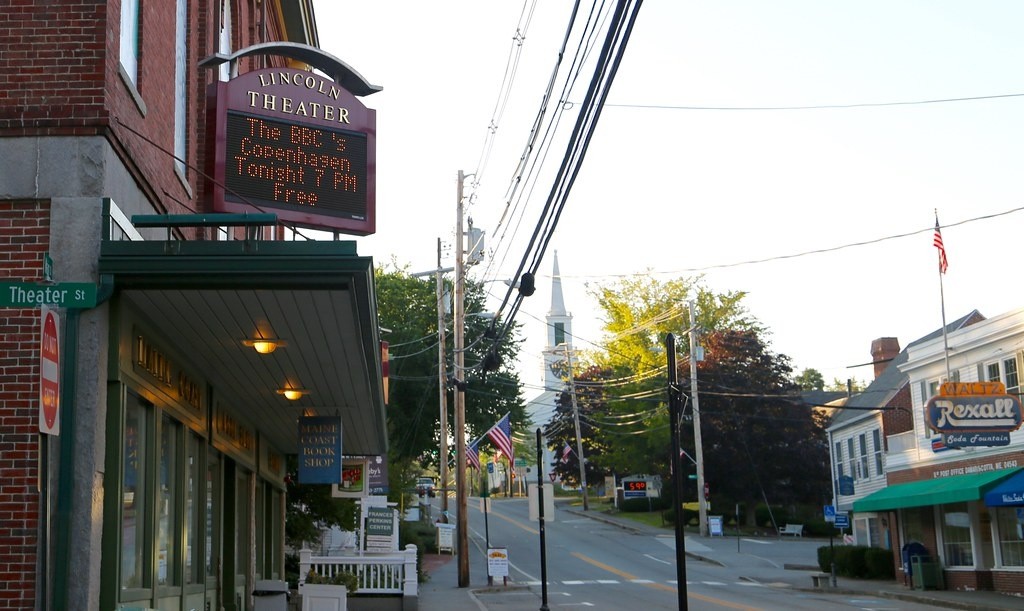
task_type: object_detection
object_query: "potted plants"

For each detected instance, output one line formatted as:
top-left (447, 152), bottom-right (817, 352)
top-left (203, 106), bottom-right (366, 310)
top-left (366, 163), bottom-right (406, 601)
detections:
top-left (301, 569), bottom-right (360, 611)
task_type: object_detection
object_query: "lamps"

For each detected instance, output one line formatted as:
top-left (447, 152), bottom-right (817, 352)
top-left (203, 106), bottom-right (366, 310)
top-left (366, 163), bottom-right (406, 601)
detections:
top-left (275, 388), bottom-right (310, 401)
top-left (241, 339), bottom-right (289, 354)
top-left (880, 516), bottom-right (886, 528)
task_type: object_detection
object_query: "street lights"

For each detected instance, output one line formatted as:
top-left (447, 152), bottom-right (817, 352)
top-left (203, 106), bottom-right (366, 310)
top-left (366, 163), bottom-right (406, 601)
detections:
top-left (439, 312), bottom-right (496, 546)
top-left (649, 346), bottom-right (708, 536)
top-left (453, 279), bottom-right (522, 589)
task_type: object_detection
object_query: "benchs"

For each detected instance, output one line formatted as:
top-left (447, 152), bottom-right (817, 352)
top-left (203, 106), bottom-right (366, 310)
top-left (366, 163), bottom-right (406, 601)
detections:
top-left (810, 573), bottom-right (830, 589)
top-left (779, 524), bottom-right (803, 538)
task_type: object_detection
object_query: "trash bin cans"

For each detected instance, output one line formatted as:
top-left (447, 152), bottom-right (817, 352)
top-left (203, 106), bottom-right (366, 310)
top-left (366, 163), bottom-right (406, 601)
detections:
top-left (254, 580), bottom-right (291, 611)
top-left (910, 554), bottom-right (938, 592)
top-left (901, 542), bottom-right (926, 588)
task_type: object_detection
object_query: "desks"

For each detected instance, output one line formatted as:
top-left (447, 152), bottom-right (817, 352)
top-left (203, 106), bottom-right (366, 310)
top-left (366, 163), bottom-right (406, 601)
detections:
top-left (435, 523), bottom-right (456, 557)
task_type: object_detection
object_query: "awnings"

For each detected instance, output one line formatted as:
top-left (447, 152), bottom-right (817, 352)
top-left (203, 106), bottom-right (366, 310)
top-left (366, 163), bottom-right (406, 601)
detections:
top-left (853, 467), bottom-right (1024, 513)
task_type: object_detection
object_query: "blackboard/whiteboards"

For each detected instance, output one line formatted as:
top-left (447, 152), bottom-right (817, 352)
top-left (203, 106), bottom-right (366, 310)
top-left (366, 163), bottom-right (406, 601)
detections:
top-left (436, 522), bottom-right (455, 549)
top-left (365, 507), bottom-right (393, 535)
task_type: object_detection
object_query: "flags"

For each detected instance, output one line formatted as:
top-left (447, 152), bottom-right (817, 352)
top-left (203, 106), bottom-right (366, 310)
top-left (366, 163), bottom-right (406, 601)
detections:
top-left (549, 469), bottom-right (557, 481)
top-left (453, 438), bottom-right (480, 473)
top-left (563, 442), bottom-right (572, 464)
top-left (934, 215), bottom-right (948, 273)
top-left (488, 415), bottom-right (514, 470)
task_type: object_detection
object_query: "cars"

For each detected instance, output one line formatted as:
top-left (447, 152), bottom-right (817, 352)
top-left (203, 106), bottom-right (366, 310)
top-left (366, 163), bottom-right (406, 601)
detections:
top-left (417, 478), bottom-right (436, 494)
